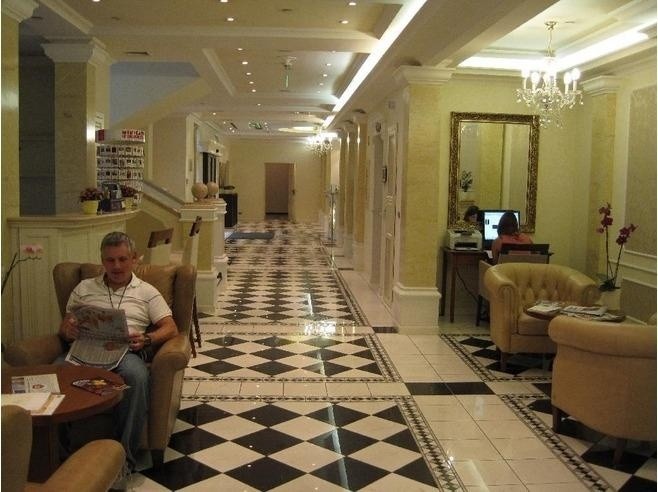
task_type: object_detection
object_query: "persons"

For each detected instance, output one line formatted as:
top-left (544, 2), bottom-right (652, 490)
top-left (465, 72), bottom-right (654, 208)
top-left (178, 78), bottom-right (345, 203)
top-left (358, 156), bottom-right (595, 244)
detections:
top-left (491, 211), bottom-right (534, 266)
top-left (52, 232), bottom-right (178, 471)
top-left (462, 205), bottom-right (480, 224)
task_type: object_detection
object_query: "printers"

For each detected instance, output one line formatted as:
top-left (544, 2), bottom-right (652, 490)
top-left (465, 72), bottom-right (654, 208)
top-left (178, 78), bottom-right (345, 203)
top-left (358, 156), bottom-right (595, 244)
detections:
top-left (447, 229), bottom-right (482, 252)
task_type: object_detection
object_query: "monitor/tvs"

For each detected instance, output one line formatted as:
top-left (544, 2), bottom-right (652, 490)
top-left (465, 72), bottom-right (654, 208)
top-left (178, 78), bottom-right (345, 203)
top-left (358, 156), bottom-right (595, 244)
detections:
top-left (483, 211), bottom-right (519, 245)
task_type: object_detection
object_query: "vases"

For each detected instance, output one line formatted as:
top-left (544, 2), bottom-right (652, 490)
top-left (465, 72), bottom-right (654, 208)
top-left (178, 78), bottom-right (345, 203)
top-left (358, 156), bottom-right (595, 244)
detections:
top-left (603, 290), bottom-right (622, 313)
top-left (82, 199), bottom-right (98, 214)
top-left (124, 196), bottom-right (134, 209)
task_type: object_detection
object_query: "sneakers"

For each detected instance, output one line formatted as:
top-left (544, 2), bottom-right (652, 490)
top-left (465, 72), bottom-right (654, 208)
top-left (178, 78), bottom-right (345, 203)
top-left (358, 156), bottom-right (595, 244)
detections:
top-left (108, 458), bottom-right (132, 492)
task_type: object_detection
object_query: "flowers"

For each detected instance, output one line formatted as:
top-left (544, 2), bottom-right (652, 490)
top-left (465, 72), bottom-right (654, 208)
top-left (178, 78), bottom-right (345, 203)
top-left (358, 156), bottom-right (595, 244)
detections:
top-left (2, 241), bottom-right (44, 298)
top-left (120, 184), bottom-right (138, 196)
top-left (594, 202), bottom-right (638, 290)
top-left (79, 186), bottom-right (100, 200)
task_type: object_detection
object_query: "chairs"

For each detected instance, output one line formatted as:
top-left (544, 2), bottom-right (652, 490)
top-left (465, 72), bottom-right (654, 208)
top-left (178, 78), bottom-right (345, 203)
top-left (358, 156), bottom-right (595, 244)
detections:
top-left (172, 216), bottom-right (206, 357)
top-left (3, 404), bottom-right (125, 491)
top-left (480, 263), bottom-right (599, 373)
top-left (477, 242), bottom-right (548, 325)
top-left (10, 260), bottom-right (199, 466)
top-left (136, 228), bottom-right (174, 264)
top-left (543, 312), bottom-right (657, 464)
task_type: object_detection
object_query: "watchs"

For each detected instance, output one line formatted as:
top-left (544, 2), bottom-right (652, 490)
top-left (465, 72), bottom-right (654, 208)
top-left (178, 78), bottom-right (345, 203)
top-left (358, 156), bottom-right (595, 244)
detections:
top-left (142, 333), bottom-right (151, 348)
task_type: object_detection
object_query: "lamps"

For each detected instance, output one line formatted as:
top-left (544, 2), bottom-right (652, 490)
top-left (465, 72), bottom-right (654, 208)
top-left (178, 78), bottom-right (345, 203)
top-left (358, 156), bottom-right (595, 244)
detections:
top-left (513, 14), bottom-right (586, 131)
top-left (306, 132), bottom-right (339, 159)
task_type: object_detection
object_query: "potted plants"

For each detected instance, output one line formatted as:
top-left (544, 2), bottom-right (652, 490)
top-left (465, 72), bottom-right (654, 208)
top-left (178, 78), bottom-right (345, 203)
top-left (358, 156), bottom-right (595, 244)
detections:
top-left (460, 167), bottom-right (475, 198)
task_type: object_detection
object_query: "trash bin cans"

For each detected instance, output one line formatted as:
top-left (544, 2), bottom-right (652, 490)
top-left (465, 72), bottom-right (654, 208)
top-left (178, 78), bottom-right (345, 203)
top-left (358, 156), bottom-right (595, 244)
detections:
top-left (219, 185), bottom-right (238, 227)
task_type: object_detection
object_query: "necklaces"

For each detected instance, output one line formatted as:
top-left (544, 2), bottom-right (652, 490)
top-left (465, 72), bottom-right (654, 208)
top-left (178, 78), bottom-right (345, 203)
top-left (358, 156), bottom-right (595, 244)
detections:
top-left (104, 277), bottom-right (131, 308)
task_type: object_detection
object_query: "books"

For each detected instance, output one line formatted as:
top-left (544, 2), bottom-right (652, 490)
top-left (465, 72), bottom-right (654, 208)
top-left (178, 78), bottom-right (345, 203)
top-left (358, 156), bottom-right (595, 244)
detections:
top-left (528, 300), bottom-right (620, 323)
top-left (2, 391), bottom-right (51, 411)
top-left (11, 372), bottom-right (61, 394)
top-left (26, 394), bottom-right (65, 415)
top-left (71, 375), bottom-right (130, 398)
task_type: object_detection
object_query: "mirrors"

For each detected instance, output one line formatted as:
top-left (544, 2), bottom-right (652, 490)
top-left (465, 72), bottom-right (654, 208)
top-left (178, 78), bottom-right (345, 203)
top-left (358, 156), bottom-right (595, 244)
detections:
top-left (448, 111), bottom-right (537, 233)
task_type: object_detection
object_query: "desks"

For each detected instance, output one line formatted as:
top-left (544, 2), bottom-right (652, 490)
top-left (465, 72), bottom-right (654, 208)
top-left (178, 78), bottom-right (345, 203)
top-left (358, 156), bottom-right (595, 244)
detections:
top-left (8, 359), bottom-right (120, 476)
top-left (440, 246), bottom-right (552, 322)
top-left (522, 298), bottom-right (624, 326)
